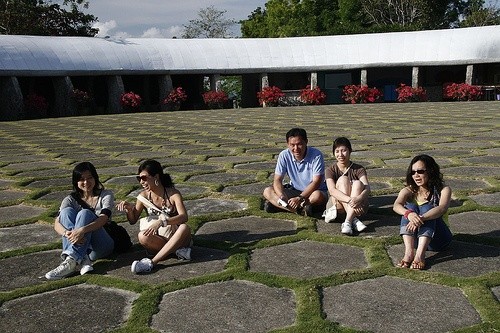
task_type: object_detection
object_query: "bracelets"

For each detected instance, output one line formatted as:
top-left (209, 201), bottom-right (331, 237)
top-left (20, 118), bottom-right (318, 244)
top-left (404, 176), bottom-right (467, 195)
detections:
top-left (298, 195), bottom-right (304, 201)
top-left (404, 209), bottom-right (414, 220)
top-left (159, 215), bottom-right (167, 228)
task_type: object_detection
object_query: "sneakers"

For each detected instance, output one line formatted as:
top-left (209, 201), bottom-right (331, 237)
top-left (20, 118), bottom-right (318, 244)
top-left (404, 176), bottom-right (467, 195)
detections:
top-left (340, 220), bottom-right (353, 235)
top-left (352, 218), bottom-right (368, 232)
top-left (45, 256), bottom-right (76, 279)
top-left (131, 257), bottom-right (153, 273)
top-left (78, 255), bottom-right (93, 276)
top-left (176, 247), bottom-right (193, 261)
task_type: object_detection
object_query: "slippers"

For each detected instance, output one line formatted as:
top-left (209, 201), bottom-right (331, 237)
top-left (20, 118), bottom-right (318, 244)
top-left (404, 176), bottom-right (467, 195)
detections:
top-left (396, 256), bottom-right (426, 269)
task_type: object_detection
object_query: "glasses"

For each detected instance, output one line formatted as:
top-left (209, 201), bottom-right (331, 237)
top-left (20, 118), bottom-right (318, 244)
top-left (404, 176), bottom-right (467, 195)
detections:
top-left (136, 175), bottom-right (148, 182)
top-left (410, 170), bottom-right (427, 175)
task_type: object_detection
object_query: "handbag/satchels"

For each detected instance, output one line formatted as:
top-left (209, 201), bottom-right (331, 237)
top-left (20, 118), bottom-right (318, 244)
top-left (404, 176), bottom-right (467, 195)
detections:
top-left (135, 193), bottom-right (179, 242)
top-left (321, 203), bottom-right (337, 223)
top-left (105, 221), bottom-right (133, 250)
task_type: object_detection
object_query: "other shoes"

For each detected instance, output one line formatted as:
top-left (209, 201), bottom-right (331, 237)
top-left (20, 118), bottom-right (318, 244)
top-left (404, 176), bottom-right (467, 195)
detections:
top-left (297, 202), bottom-right (313, 216)
top-left (264, 200), bottom-right (282, 213)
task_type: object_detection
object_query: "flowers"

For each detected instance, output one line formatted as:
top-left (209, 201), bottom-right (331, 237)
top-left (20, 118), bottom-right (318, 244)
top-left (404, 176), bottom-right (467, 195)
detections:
top-left (300, 84), bottom-right (325, 102)
top-left (119, 92), bottom-right (141, 105)
top-left (443, 83), bottom-right (485, 101)
top-left (202, 89), bottom-right (227, 103)
top-left (26, 95), bottom-right (46, 110)
top-left (397, 83), bottom-right (428, 102)
top-left (163, 87), bottom-right (187, 102)
top-left (258, 86), bottom-right (285, 101)
top-left (67, 89), bottom-right (94, 104)
top-left (342, 84), bottom-right (384, 103)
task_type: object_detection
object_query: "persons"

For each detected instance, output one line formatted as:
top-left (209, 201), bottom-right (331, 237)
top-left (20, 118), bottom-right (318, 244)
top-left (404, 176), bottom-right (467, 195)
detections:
top-left (45, 162), bottom-right (114, 282)
top-left (325, 137), bottom-right (370, 234)
top-left (393, 154), bottom-right (451, 269)
top-left (263, 128), bottom-right (328, 217)
top-left (115, 159), bottom-right (192, 274)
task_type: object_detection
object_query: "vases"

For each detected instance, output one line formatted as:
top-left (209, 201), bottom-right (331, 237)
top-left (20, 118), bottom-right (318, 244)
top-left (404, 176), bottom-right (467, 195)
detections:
top-left (127, 107), bottom-right (142, 112)
top-left (356, 97), bottom-right (367, 103)
top-left (267, 102), bottom-right (278, 107)
top-left (308, 100), bottom-right (316, 105)
top-left (170, 104), bottom-right (180, 110)
top-left (210, 103), bottom-right (223, 109)
top-left (407, 96), bottom-right (419, 102)
top-left (458, 96), bottom-right (469, 101)
